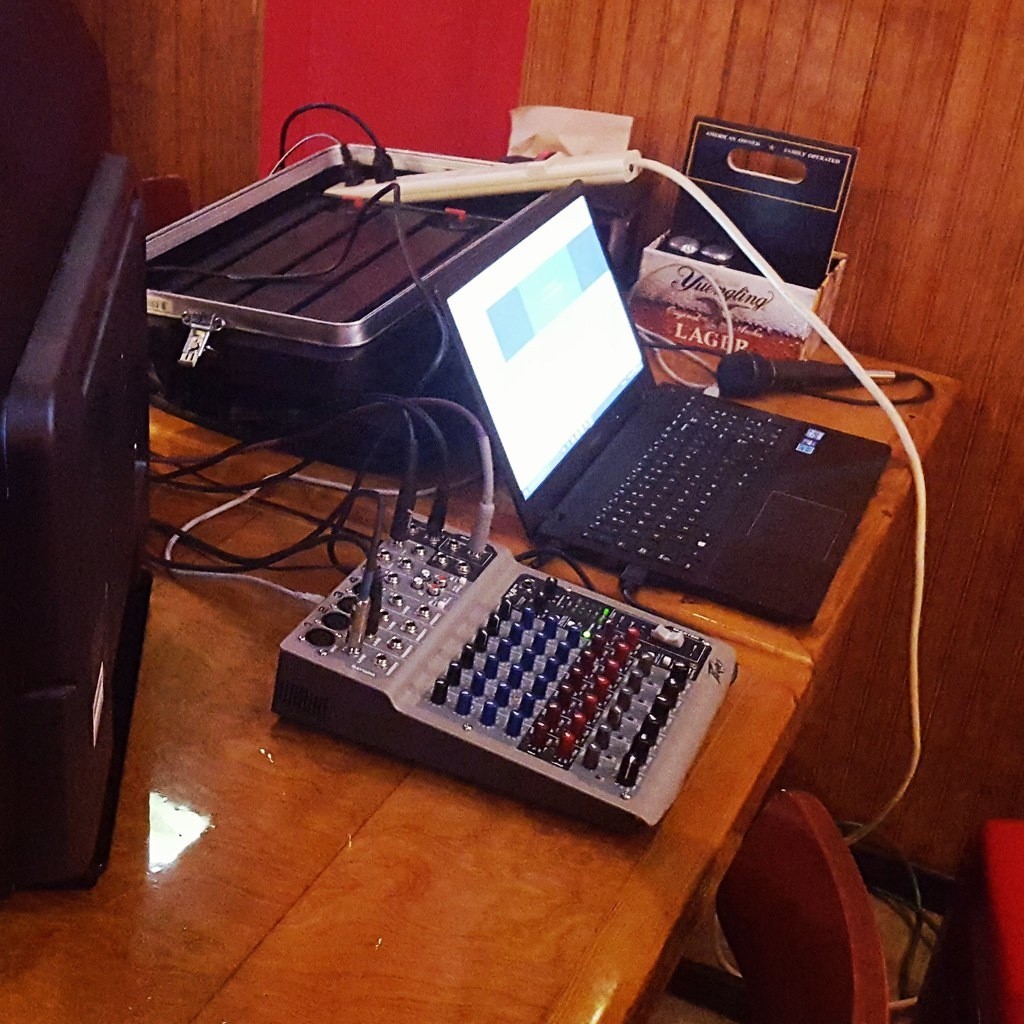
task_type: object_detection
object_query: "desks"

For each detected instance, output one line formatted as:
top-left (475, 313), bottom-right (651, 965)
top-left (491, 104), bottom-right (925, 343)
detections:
top-left (0, 296), bottom-right (963, 1024)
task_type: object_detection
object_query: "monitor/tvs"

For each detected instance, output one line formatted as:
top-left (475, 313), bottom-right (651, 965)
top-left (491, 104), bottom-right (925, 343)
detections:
top-left (0, 151), bottom-right (157, 897)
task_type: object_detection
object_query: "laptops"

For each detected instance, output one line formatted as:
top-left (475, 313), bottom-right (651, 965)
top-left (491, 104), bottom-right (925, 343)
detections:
top-left (423, 177), bottom-right (894, 628)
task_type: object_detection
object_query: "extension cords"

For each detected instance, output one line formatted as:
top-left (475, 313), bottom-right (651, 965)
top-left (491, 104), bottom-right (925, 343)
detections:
top-left (322, 148), bottom-right (645, 204)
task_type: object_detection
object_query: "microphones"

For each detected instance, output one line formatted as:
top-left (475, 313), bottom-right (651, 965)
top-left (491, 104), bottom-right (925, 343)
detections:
top-left (716, 349), bottom-right (914, 400)
